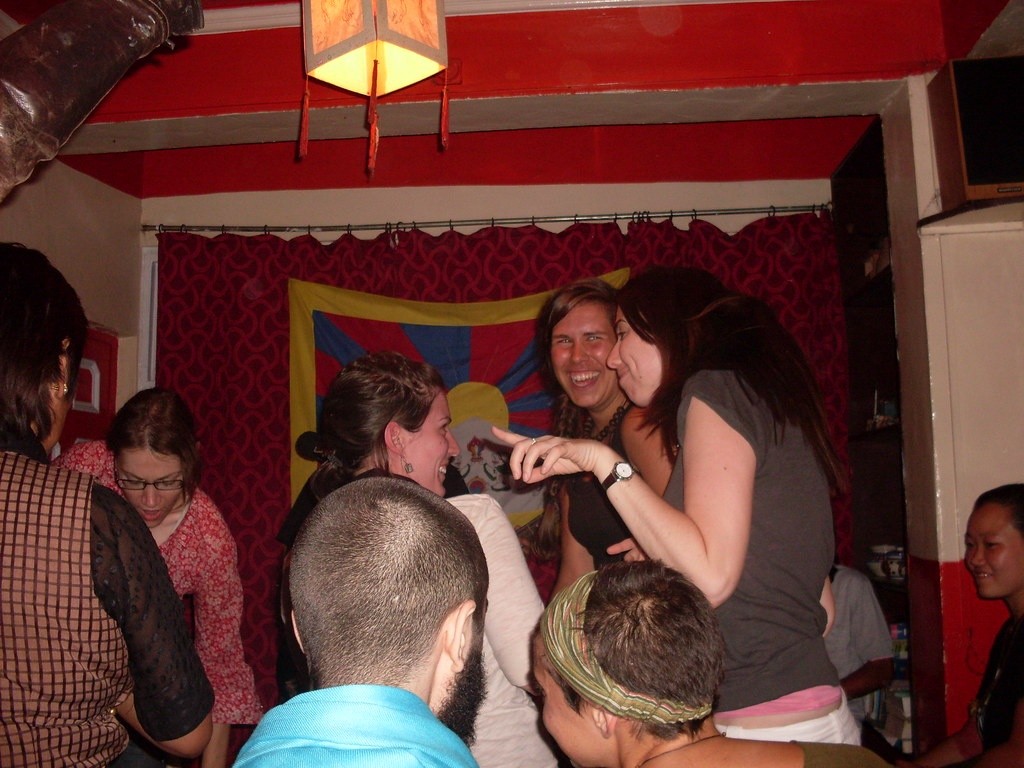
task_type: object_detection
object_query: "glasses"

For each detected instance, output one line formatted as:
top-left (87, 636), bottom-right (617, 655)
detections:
top-left (113, 469), bottom-right (183, 491)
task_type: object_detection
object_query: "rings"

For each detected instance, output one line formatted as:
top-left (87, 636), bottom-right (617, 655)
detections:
top-left (532, 438), bottom-right (536, 445)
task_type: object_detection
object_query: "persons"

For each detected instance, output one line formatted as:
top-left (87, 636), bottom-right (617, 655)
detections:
top-left (0, 240), bottom-right (1024, 768)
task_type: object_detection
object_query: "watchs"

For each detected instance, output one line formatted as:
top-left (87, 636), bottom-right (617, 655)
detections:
top-left (602, 461), bottom-right (634, 491)
